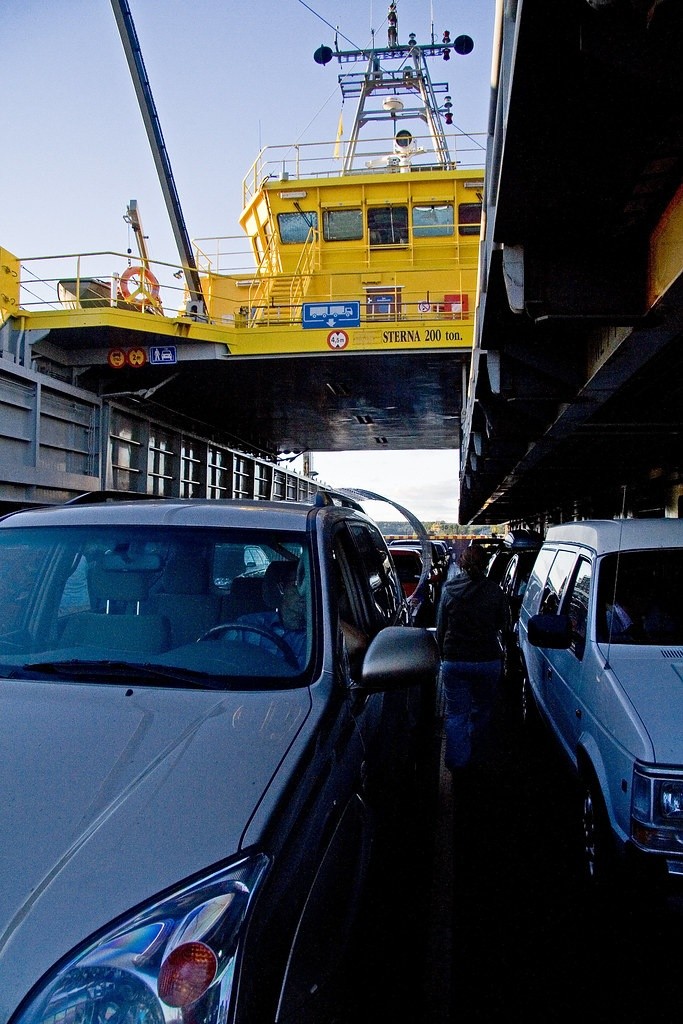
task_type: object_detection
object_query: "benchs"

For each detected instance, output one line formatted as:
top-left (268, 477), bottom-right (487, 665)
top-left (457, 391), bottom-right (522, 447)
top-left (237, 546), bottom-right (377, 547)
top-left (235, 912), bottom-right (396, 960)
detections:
top-left (149, 576), bottom-right (267, 642)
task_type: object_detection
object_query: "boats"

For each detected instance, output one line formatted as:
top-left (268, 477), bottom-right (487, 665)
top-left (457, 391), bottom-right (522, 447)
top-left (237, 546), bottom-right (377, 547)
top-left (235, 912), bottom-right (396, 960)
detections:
top-left (59, 274), bottom-right (165, 315)
top-left (56, 277), bottom-right (148, 313)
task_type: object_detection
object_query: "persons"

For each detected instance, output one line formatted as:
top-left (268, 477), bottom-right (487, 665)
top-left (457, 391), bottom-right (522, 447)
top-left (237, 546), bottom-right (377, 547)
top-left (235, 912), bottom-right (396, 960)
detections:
top-left (606, 570), bottom-right (673, 635)
top-left (437, 545), bottom-right (518, 781)
top-left (222, 559), bottom-right (350, 685)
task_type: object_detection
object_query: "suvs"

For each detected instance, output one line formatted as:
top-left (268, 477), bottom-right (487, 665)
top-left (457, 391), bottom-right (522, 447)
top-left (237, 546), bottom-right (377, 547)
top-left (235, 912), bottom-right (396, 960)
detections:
top-left (515, 518), bottom-right (683, 887)
top-left (211, 544), bottom-right (271, 593)
top-left (0, 489), bottom-right (443, 1024)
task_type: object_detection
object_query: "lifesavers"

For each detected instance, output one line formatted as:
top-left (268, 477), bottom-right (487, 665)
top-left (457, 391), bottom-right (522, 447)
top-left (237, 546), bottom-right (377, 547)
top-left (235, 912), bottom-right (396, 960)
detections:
top-left (119, 266), bottom-right (160, 305)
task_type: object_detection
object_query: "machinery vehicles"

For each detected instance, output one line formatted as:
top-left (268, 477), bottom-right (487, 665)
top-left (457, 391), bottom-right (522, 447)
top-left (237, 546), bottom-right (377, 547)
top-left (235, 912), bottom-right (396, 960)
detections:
top-left (123, 201), bottom-right (161, 313)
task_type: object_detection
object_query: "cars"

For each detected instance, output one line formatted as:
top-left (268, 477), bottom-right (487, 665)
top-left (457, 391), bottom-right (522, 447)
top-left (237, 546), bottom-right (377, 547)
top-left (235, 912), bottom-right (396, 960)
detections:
top-left (469, 530), bottom-right (544, 686)
top-left (388, 539), bottom-right (453, 624)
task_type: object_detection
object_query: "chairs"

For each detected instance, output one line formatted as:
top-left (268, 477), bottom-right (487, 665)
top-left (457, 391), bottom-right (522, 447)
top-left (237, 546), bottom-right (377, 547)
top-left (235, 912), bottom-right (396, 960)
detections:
top-left (56, 571), bottom-right (171, 656)
